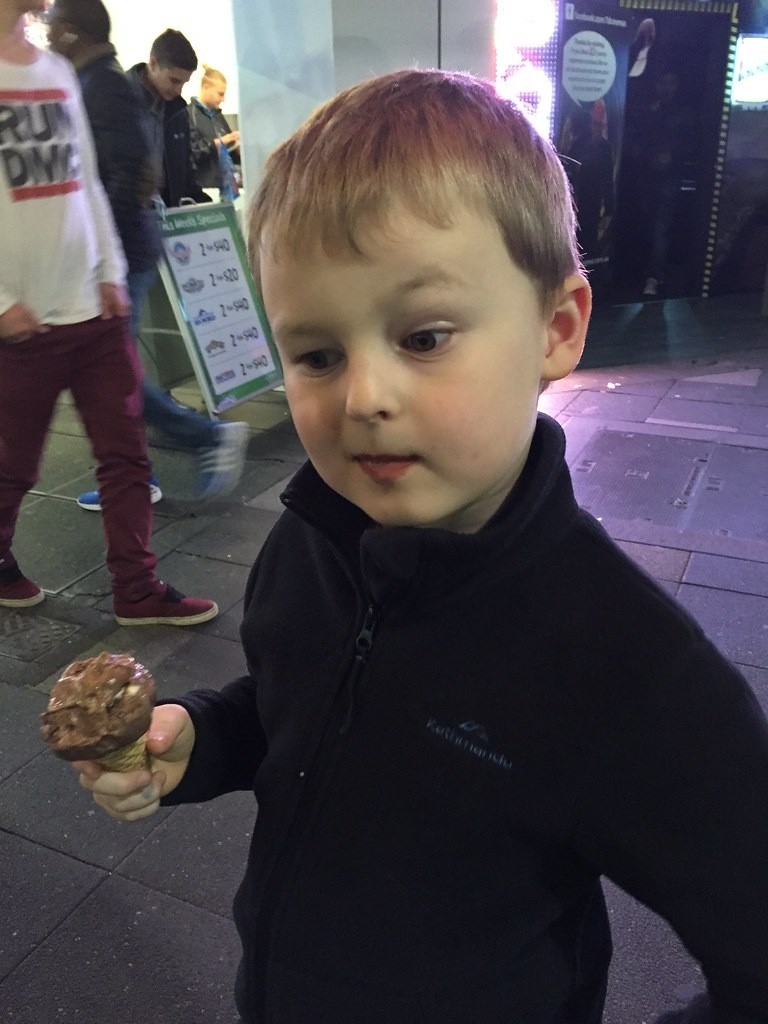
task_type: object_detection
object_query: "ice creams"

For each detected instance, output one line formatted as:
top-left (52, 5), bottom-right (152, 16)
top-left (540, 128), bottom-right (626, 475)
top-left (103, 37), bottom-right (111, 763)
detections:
top-left (40, 652), bottom-right (154, 774)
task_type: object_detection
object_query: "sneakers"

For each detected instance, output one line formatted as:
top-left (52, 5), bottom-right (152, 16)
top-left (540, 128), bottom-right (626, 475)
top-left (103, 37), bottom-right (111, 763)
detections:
top-left (0, 550), bottom-right (45, 607)
top-left (195, 422), bottom-right (249, 496)
top-left (113, 580), bottom-right (218, 625)
top-left (77, 474), bottom-right (163, 511)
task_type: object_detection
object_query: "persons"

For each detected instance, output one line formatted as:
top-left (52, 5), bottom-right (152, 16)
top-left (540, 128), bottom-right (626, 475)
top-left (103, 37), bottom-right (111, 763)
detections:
top-left (1, 0), bottom-right (218, 626)
top-left (123, 28), bottom-right (212, 206)
top-left (45, 0), bottom-right (250, 510)
top-left (186, 69), bottom-right (242, 188)
top-left (65, 66), bottom-right (768, 1023)
